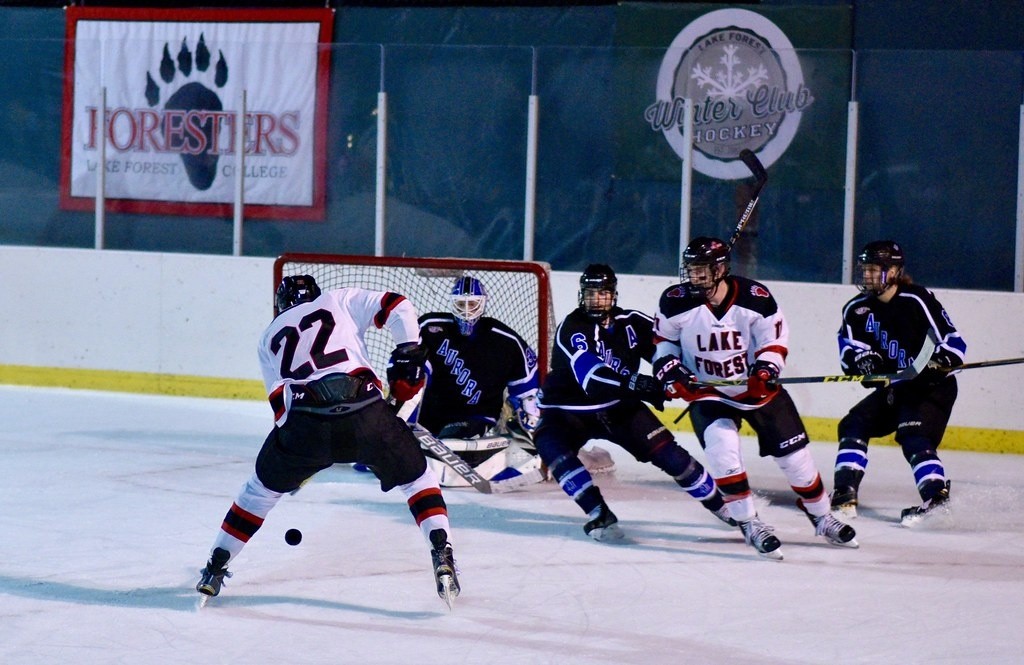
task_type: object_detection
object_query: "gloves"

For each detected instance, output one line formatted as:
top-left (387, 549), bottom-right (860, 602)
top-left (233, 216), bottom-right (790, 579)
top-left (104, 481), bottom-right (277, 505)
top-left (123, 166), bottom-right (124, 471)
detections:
top-left (621, 372), bottom-right (672, 413)
top-left (654, 359), bottom-right (701, 401)
top-left (854, 349), bottom-right (884, 376)
top-left (921, 360), bottom-right (944, 377)
top-left (747, 361), bottom-right (777, 398)
top-left (387, 348), bottom-right (430, 401)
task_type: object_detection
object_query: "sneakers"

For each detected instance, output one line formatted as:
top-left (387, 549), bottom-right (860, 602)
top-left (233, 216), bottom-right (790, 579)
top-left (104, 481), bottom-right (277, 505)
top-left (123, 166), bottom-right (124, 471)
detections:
top-left (197, 547), bottom-right (234, 607)
top-left (796, 498), bottom-right (860, 549)
top-left (899, 479), bottom-right (951, 528)
top-left (712, 500), bottom-right (739, 527)
top-left (741, 511), bottom-right (785, 561)
top-left (577, 486), bottom-right (625, 541)
top-left (430, 528), bottom-right (462, 610)
top-left (827, 487), bottom-right (857, 518)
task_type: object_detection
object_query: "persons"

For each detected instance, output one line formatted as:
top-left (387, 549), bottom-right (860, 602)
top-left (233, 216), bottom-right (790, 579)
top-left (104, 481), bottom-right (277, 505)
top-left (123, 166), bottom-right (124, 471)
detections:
top-left (652, 236), bottom-right (859, 560)
top-left (827, 239), bottom-right (968, 526)
top-left (530, 261), bottom-right (739, 545)
top-left (351, 275), bottom-right (544, 492)
top-left (194, 275), bottom-right (461, 612)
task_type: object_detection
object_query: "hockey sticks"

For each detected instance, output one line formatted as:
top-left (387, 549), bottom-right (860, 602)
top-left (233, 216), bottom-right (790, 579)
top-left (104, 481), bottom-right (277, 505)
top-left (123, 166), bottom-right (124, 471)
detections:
top-left (688, 356), bottom-right (1024, 386)
top-left (663, 147), bottom-right (770, 393)
top-left (406, 421), bottom-right (548, 496)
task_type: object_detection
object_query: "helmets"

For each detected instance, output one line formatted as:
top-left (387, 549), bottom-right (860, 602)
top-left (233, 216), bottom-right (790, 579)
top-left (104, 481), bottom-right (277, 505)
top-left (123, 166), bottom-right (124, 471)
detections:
top-left (451, 277), bottom-right (488, 336)
top-left (277, 275), bottom-right (321, 313)
top-left (680, 236), bottom-right (731, 300)
top-left (853, 240), bottom-right (903, 297)
top-left (577, 264), bottom-right (617, 319)
